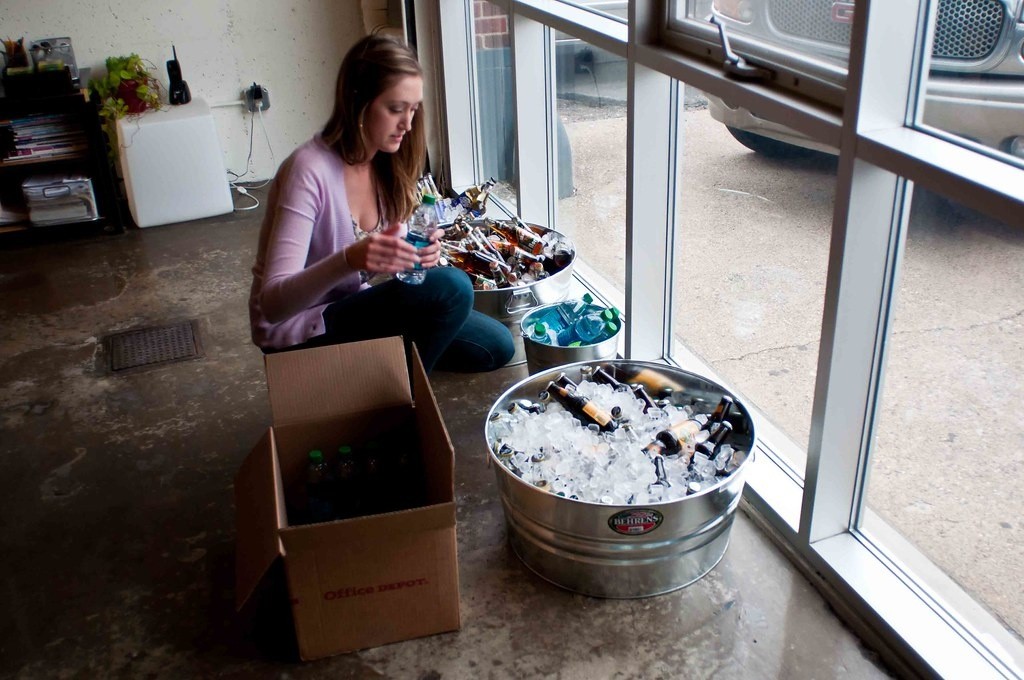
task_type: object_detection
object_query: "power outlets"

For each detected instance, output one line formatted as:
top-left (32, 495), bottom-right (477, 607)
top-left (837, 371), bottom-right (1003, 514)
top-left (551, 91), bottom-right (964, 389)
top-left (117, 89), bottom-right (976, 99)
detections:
top-left (243, 87), bottom-right (270, 111)
top-left (575, 51), bottom-right (594, 73)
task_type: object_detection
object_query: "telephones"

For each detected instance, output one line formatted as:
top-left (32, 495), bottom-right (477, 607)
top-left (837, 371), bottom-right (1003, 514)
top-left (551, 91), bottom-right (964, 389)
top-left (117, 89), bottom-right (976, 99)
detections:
top-left (165, 45), bottom-right (192, 105)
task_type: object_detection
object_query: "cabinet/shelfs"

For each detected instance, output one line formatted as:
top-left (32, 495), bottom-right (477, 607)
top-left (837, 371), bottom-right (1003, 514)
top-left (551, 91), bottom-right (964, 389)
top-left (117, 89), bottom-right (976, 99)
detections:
top-left (0, 96), bottom-right (124, 256)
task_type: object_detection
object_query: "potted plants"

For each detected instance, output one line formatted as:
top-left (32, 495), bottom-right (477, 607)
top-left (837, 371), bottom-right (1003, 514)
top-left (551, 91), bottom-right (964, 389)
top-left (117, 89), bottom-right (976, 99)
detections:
top-left (89, 54), bottom-right (164, 125)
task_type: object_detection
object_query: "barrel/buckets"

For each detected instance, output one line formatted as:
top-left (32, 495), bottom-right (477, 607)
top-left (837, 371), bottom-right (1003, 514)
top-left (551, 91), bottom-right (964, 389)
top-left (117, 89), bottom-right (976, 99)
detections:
top-left (520, 299), bottom-right (620, 381)
top-left (404, 216), bottom-right (574, 369)
top-left (484, 358), bottom-right (756, 598)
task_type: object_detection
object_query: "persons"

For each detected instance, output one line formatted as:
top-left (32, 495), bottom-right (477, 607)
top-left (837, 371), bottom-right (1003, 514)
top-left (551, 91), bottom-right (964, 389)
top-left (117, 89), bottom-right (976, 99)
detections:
top-left (248, 33), bottom-right (515, 375)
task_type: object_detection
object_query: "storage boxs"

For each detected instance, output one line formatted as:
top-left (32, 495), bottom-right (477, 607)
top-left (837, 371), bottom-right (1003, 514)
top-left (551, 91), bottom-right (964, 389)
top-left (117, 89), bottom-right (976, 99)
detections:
top-left (230, 336), bottom-right (463, 663)
top-left (19, 178), bottom-right (101, 226)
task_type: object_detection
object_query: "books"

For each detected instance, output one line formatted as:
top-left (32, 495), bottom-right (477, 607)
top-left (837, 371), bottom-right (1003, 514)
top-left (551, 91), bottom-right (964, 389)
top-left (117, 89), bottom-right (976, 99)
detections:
top-left (0, 113), bottom-right (88, 161)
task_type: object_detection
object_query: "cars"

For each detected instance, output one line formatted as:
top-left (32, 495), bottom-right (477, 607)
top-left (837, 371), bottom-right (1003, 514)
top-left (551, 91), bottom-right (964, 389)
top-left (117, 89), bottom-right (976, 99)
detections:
top-left (706, 0), bottom-right (1024, 153)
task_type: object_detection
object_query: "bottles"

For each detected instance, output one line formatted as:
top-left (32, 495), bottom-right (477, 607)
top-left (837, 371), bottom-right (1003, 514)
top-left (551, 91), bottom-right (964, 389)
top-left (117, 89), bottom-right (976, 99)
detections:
top-left (333, 445), bottom-right (358, 487)
top-left (302, 451), bottom-right (333, 497)
top-left (396, 195), bottom-right (437, 285)
top-left (407, 169), bottom-right (572, 291)
top-left (489, 363), bottom-right (751, 506)
top-left (525, 293), bottom-right (620, 346)
